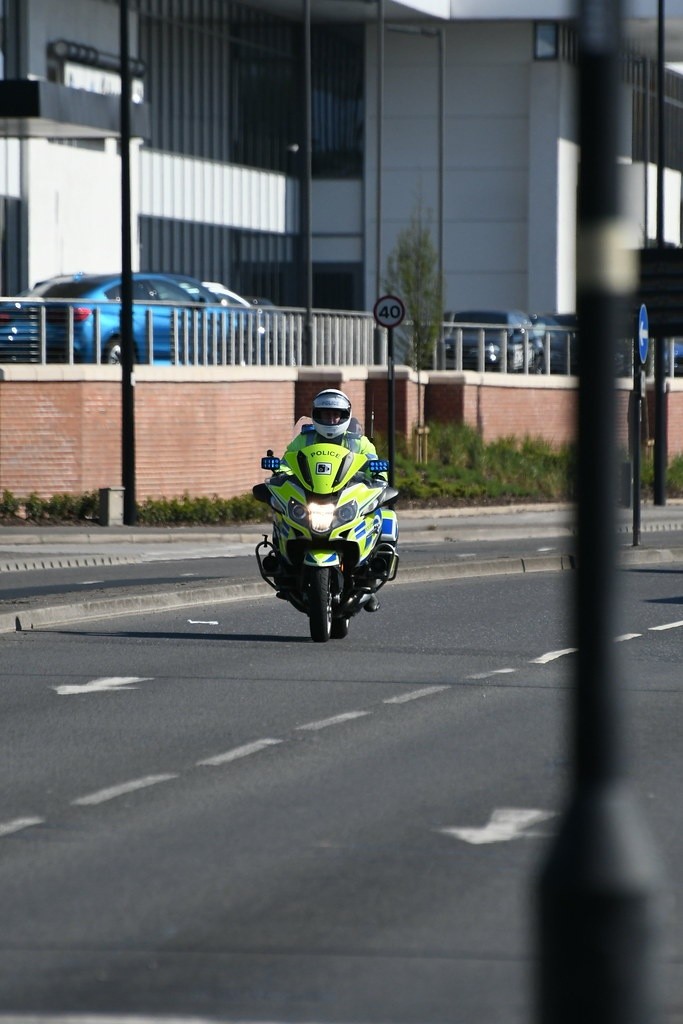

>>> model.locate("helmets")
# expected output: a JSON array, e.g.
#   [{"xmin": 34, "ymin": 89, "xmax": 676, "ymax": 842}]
[{"xmin": 311, "ymin": 389, "xmax": 351, "ymax": 440}]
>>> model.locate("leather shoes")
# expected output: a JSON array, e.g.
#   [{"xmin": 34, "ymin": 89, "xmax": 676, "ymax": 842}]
[
  {"xmin": 364, "ymin": 592, "xmax": 380, "ymax": 612},
  {"xmin": 263, "ymin": 556, "xmax": 280, "ymax": 585}
]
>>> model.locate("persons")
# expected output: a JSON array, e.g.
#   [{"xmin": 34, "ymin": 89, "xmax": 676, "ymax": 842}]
[{"xmin": 271, "ymin": 389, "xmax": 382, "ymax": 613}]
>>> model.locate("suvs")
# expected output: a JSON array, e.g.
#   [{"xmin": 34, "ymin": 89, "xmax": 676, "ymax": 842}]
[{"xmin": 430, "ymin": 306, "xmax": 546, "ymax": 378}]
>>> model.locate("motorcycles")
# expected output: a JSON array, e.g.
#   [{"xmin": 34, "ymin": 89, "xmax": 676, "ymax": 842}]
[{"xmin": 250, "ymin": 453, "xmax": 400, "ymax": 642}]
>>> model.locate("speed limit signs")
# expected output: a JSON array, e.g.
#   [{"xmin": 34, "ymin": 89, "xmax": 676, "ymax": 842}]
[{"xmin": 373, "ymin": 294, "xmax": 406, "ymax": 328}]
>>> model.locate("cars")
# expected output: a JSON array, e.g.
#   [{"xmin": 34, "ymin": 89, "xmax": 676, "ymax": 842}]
[
  {"xmin": 1, "ymin": 271, "xmax": 236, "ymax": 366},
  {"xmin": 164, "ymin": 271, "xmax": 309, "ymax": 368},
  {"xmin": 526, "ymin": 313, "xmax": 683, "ymax": 379}
]
